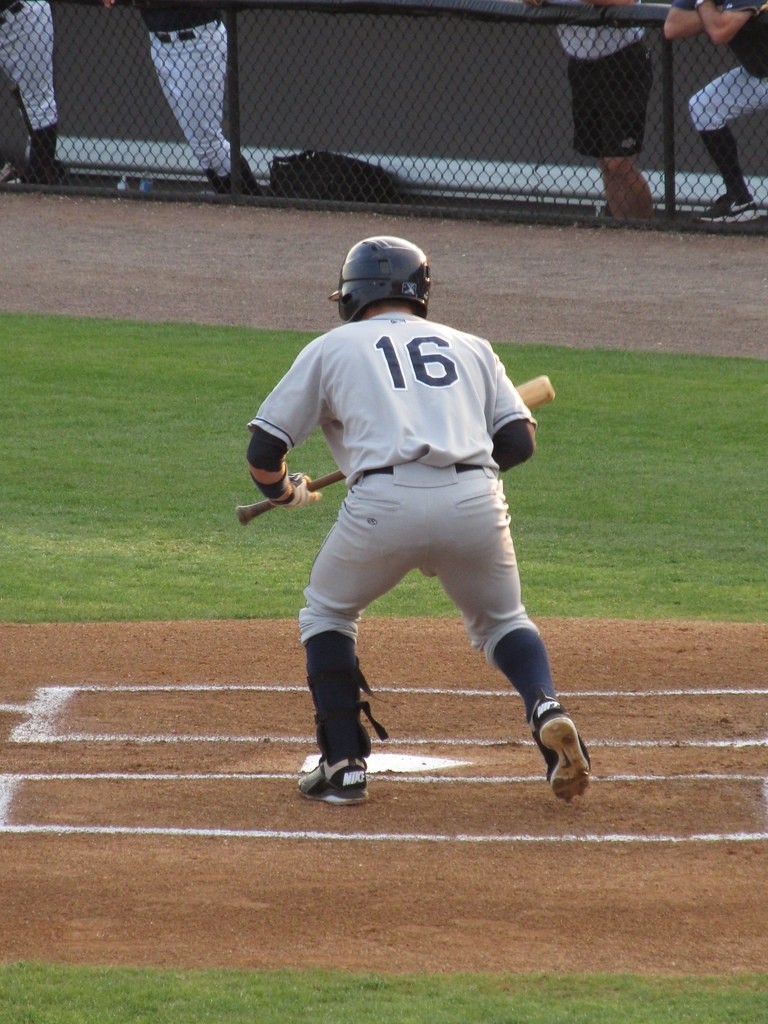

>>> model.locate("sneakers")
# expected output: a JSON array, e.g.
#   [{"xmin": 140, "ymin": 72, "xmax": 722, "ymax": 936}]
[
  {"xmin": 298, "ymin": 753, "xmax": 369, "ymax": 805},
  {"xmin": 696, "ymin": 192, "xmax": 760, "ymax": 222},
  {"xmin": 531, "ymin": 688, "xmax": 590, "ymax": 801}
]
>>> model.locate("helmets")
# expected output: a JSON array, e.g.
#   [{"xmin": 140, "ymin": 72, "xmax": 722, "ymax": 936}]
[{"xmin": 328, "ymin": 235, "xmax": 431, "ymax": 324}]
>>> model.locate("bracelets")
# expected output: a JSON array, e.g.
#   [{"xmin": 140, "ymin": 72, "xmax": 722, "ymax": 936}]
[{"xmin": 250, "ymin": 465, "xmax": 291, "ymax": 501}]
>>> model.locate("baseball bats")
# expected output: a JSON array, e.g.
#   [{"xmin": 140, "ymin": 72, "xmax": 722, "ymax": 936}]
[{"xmin": 236, "ymin": 373, "xmax": 557, "ymax": 515}]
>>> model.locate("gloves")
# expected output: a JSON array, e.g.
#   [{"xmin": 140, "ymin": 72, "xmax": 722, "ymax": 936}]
[
  {"xmin": 672, "ymin": 0, "xmax": 705, "ymax": 10},
  {"xmin": 269, "ymin": 472, "xmax": 323, "ymax": 510}
]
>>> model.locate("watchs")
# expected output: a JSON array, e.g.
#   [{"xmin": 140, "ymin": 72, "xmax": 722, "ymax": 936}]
[{"xmin": 694, "ymin": 0, "xmax": 704, "ymax": 9}]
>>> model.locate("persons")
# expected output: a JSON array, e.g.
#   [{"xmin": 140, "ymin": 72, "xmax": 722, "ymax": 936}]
[
  {"xmin": 0, "ymin": 0, "xmax": 65, "ymax": 191},
  {"xmin": 247, "ymin": 234, "xmax": 593, "ymax": 806},
  {"xmin": 104, "ymin": 1, "xmax": 265, "ymax": 197},
  {"xmin": 522, "ymin": 0, "xmax": 768, "ymax": 227}
]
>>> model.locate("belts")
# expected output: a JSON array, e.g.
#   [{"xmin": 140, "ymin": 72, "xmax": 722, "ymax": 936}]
[
  {"xmin": 158, "ymin": 20, "xmax": 222, "ymax": 43},
  {"xmin": 363, "ymin": 462, "xmax": 483, "ymax": 478},
  {"xmin": 0, "ymin": 2, "xmax": 24, "ymax": 25}
]
